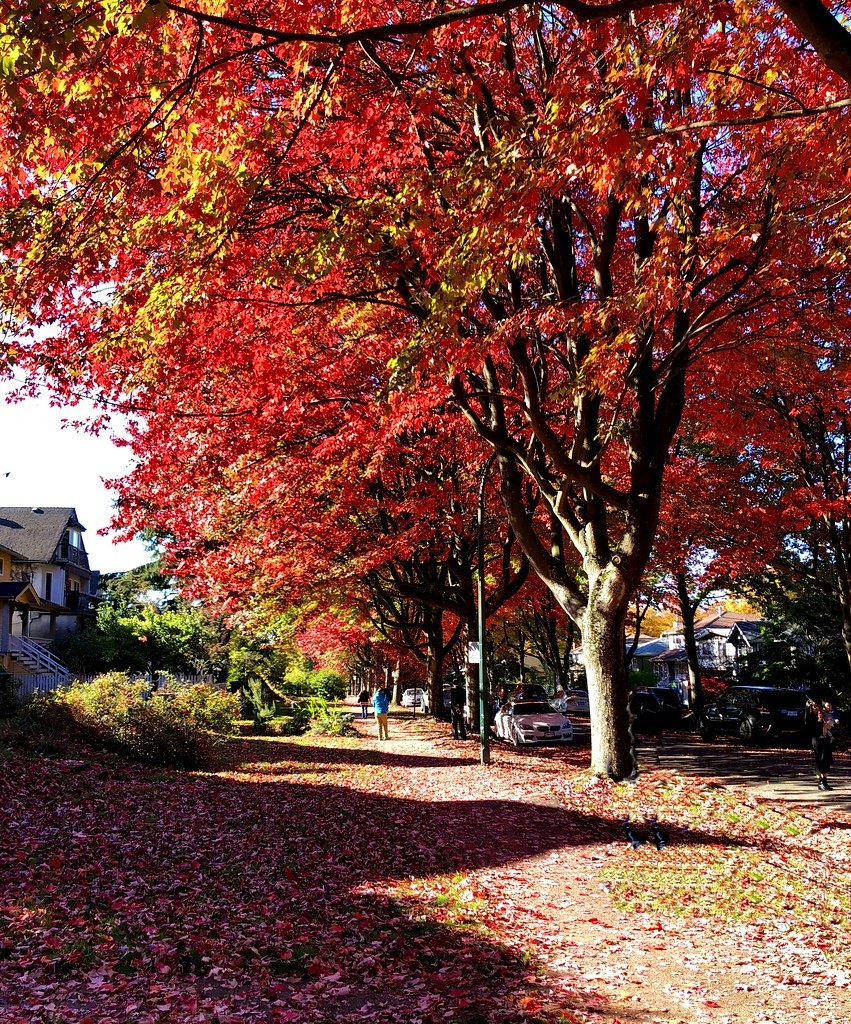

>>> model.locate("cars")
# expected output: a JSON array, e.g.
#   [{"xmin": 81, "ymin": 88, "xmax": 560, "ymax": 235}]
[
  {"xmin": 548, "ymin": 690, "xmax": 589, "ymax": 715},
  {"xmin": 636, "ymin": 687, "xmax": 684, "ymax": 719},
  {"xmin": 424, "ymin": 685, "xmax": 452, "ymax": 713},
  {"xmin": 401, "ymin": 688, "xmax": 424, "ymax": 707},
  {"xmin": 493, "ymin": 699, "xmax": 573, "ymax": 747}
]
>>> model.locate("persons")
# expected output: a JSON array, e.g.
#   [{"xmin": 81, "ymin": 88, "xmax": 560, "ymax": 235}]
[
  {"xmin": 819, "ymin": 699, "xmax": 841, "ymax": 744},
  {"xmin": 449, "ymin": 678, "xmax": 466, "ymax": 740},
  {"xmin": 805, "ymin": 699, "xmax": 835, "ymax": 791},
  {"xmin": 357, "ymin": 685, "xmax": 372, "ymax": 719},
  {"xmin": 371, "ymin": 687, "xmax": 390, "ymax": 741},
  {"xmin": 378, "ymin": 682, "xmax": 390, "ymax": 716}
]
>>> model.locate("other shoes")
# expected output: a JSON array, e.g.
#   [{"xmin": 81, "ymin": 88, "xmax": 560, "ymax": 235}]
[
  {"xmin": 385, "ymin": 737, "xmax": 389, "ymax": 740},
  {"xmin": 819, "ymin": 735, "xmax": 826, "ymax": 739},
  {"xmin": 824, "ymin": 783, "xmax": 833, "ymax": 790},
  {"xmin": 830, "ymin": 737, "xmax": 834, "ymax": 743},
  {"xmin": 818, "ymin": 784, "xmax": 827, "ymax": 791},
  {"xmin": 379, "ymin": 738, "xmax": 382, "ymax": 740}
]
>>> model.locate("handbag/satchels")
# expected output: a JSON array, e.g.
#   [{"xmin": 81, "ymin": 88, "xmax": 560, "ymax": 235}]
[{"xmin": 358, "ymin": 697, "xmax": 361, "ymax": 702}]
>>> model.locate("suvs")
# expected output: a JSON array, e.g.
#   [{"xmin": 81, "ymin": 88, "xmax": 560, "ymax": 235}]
[
  {"xmin": 490, "ymin": 684, "xmax": 516, "ymax": 709},
  {"xmin": 508, "ymin": 683, "xmax": 548, "ymax": 702},
  {"xmin": 699, "ymin": 686, "xmax": 814, "ymax": 746}
]
